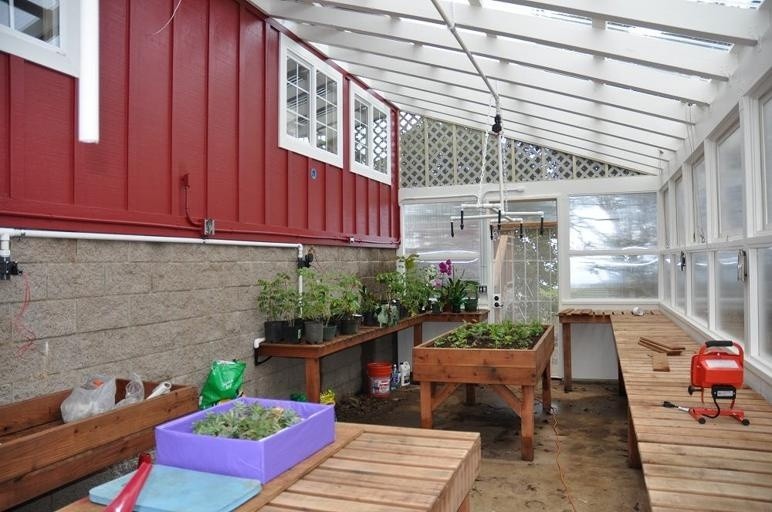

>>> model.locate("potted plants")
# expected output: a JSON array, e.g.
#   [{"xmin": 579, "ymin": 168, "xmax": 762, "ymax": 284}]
[
  {"xmin": 412, "ymin": 318, "xmax": 556, "ymax": 463},
  {"xmin": 261, "ymin": 253, "xmax": 481, "ymax": 343}
]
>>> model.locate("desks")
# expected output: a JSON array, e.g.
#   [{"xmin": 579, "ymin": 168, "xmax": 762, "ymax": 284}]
[
  {"xmin": 260, "ymin": 307, "xmax": 490, "ymax": 402},
  {"xmin": 557, "ymin": 306, "xmax": 771, "ymax": 512},
  {"xmin": 54, "ymin": 421, "xmax": 483, "ymax": 512}
]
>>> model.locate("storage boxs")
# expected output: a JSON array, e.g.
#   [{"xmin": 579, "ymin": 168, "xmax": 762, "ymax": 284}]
[{"xmin": 0, "ymin": 378, "xmax": 199, "ymax": 512}]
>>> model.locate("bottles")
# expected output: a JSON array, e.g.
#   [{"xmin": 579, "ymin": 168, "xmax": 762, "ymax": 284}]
[{"xmin": 391, "ymin": 363, "xmax": 402, "ymax": 390}]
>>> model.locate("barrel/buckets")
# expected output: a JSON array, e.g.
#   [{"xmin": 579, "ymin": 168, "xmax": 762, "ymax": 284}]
[
  {"xmin": 367, "ymin": 361, "xmax": 395, "ymax": 399},
  {"xmin": 399, "ymin": 361, "xmax": 411, "ymax": 386},
  {"xmin": 390, "ymin": 364, "xmax": 399, "ymax": 386}
]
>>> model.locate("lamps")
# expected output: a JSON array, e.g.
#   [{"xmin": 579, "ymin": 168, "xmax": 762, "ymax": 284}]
[{"xmin": 448, "ymin": 93, "xmax": 545, "ymax": 241}]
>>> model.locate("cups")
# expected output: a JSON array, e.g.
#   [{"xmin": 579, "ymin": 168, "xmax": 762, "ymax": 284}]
[{"xmin": 632, "ymin": 306, "xmax": 643, "ymax": 316}]
